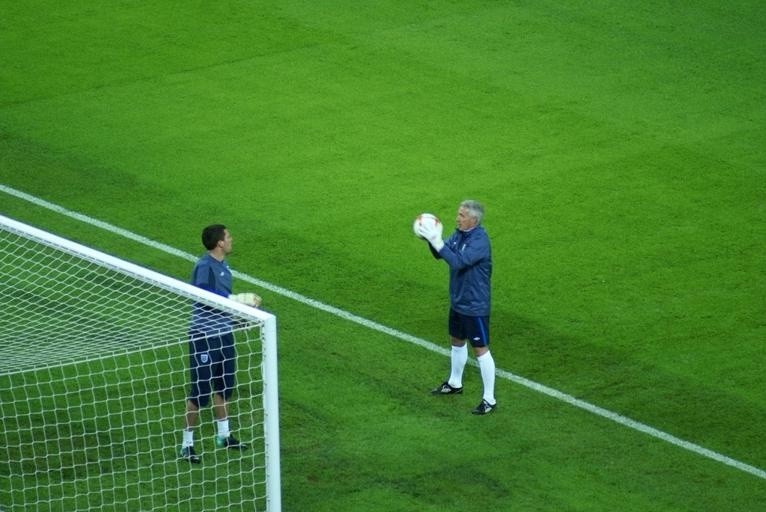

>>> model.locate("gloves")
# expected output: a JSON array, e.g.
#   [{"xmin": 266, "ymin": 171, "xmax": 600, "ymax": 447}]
[
  {"xmin": 228, "ymin": 292, "xmax": 262, "ymax": 308},
  {"xmin": 417, "ymin": 221, "xmax": 445, "ymax": 253}
]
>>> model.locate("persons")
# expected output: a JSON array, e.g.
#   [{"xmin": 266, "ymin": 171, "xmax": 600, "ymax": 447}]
[
  {"xmin": 179, "ymin": 224, "xmax": 262, "ymax": 463},
  {"xmin": 418, "ymin": 199, "xmax": 498, "ymax": 415}
]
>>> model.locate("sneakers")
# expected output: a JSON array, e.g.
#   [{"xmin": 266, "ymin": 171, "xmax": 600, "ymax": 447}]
[
  {"xmin": 179, "ymin": 445, "xmax": 201, "ymax": 465},
  {"xmin": 472, "ymin": 399, "xmax": 497, "ymax": 416},
  {"xmin": 430, "ymin": 381, "xmax": 463, "ymax": 396},
  {"xmin": 216, "ymin": 434, "xmax": 249, "ymax": 452}
]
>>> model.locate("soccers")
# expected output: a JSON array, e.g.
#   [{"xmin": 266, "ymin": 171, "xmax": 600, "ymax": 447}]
[{"xmin": 413, "ymin": 213, "xmax": 441, "ymax": 240}]
